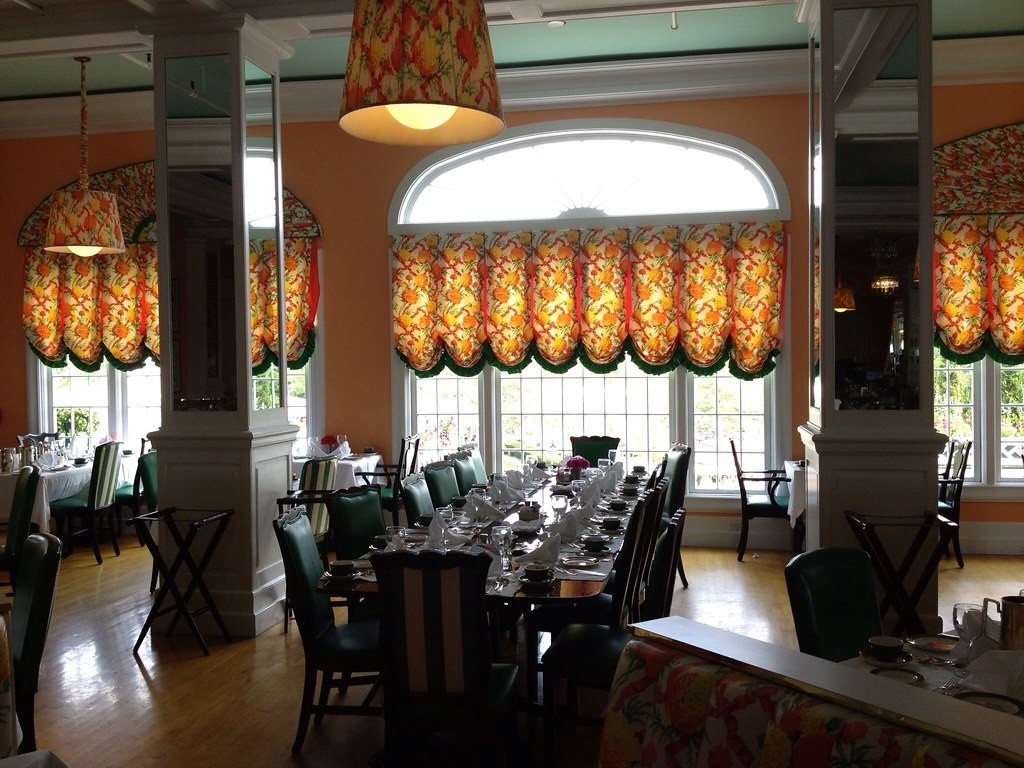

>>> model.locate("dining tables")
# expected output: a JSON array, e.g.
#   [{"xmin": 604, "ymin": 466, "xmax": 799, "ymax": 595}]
[
  {"xmin": 783, "ymin": 458, "xmax": 806, "ymax": 526},
  {"xmin": 840, "ymin": 619, "xmax": 1024, "ymax": 717},
  {"xmin": 0, "ymin": 449, "xmax": 142, "ymax": 532},
  {"xmin": 351, "ymin": 468, "xmax": 646, "ymax": 748},
  {"xmin": 292, "ymin": 452, "xmax": 384, "ymax": 487}
]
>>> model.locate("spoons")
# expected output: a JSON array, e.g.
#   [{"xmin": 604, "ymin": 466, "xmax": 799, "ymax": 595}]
[{"xmin": 497, "ymin": 578, "xmax": 509, "ymax": 592}]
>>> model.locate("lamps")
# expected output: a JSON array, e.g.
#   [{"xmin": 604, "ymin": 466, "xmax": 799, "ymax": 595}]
[
  {"xmin": 337, "ymin": 0, "xmax": 506, "ymax": 145},
  {"xmin": 44, "ymin": 56, "xmax": 125, "ymax": 256},
  {"xmin": 871, "ymin": 273, "xmax": 899, "ymax": 294},
  {"xmin": 835, "ymin": 277, "xmax": 856, "ymax": 313}
]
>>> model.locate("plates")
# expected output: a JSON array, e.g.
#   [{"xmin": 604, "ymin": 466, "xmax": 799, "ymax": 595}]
[
  {"xmin": 518, "ymin": 574, "xmax": 555, "ymax": 586},
  {"xmin": 413, "ymin": 522, "xmax": 433, "ymax": 529},
  {"xmin": 620, "ymin": 494, "xmax": 639, "ymax": 499},
  {"xmin": 362, "ymin": 452, "xmax": 377, "ymax": 456},
  {"xmin": 560, "ymin": 556, "xmax": 598, "ymax": 567},
  {"xmin": 871, "ymin": 667, "xmax": 923, "ymax": 684},
  {"xmin": 599, "ymin": 527, "xmax": 625, "ymax": 533},
  {"xmin": 862, "ymin": 651, "xmax": 914, "ymax": 668},
  {"xmin": 954, "ymin": 693, "xmax": 1024, "ymax": 717},
  {"xmin": 579, "ymin": 547, "xmax": 610, "ymax": 554},
  {"xmin": 608, "ymin": 507, "xmax": 629, "ymax": 513},
  {"xmin": 909, "ymin": 636, "xmax": 960, "ymax": 651}
]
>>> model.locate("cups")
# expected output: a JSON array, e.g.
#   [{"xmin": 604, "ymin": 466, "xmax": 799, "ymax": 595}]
[
  {"xmin": 525, "ymin": 563, "xmax": 553, "ymax": 581},
  {"xmin": 585, "ymin": 540, "xmax": 605, "ymax": 552},
  {"xmin": 626, "ymin": 476, "xmax": 638, "ymax": 484},
  {"xmin": 603, "ymin": 519, "xmax": 621, "ymax": 531},
  {"xmin": 611, "ymin": 502, "xmax": 626, "ymax": 511},
  {"xmin": 623, "ymin": 488, "xmax": 637, "ymax": 495},
  {"xmin": 420, "ymin": 514, "xmax": 433, "ymax": 526},
  {"xmin": 374, "ymin": 535, "xmax": 386, "ymax": 548},
  {"xmin": 869, "ymin": 637, "xmax": 903, "ymax": 658},
  {"xmin": 452, "ymin": 497, "xmax": 467, "ymax": 509},
  {"xmin": 75, "ymin": 458, "xmax": 85, "ymax": 464},
  {"xmin": 633, "ymin": 466, "xmax": 644, "ymax": 473},
  {"xmin": 364, "ymin": 447, "xmax": 374, "ymax": 452},
  {"xmin": 329, "ymin": 560, "xmax": 354, "ymax": 576},
  {"xmin": 535, "ymin": 462, "xmax": 546, "ymax": 470},
  {"xmin": 984, "ymin": 596, "xmax": 1024, "ymax": 651}
]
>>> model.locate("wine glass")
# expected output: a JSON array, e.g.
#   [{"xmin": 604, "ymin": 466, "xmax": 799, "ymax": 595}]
[
  {"xmin": 553, "ymin": 495, "xmax": 568, "ymax": 521},
  {"xmin": 572, "ymin": 479, "xmax": 586, "ymax": 509},
  {"xmin": 954, "ymin": 604, "xmax": 986, "ymax": 676},
  {"xmin": 598, "ymin": 460, "xmax": 611, "ymax": 477},
  {"xmin": 436, "ymin": 507, "xmax": 454, "ymax": 544},
  {"xmin": 308, "ymin": 436, "xmax": 318, "ymax": 459},
  {"xmin": 492, "ymin": 526, "xmax": 511, "ymax": 569},
  {"xmin": 493, "ymin": 474, "xmax": 507, "ymax": 508},
  {"xmin": 608, "ymin": 450, "xmax": 621, "ymax": 465},
  {"xmin": 337, "ymin": 435, "xmax": 346, "ymax": 446},
  {"xmin": 384, "ymin": 526, "xmax": 406, "ymax": 551},
  {"xmin": 517, "ymin": 464, "xmax": 529, "ymax": 495},
  {"xmin": 471, "ymin": 489, "xmax": 486, "ymax": 527}
]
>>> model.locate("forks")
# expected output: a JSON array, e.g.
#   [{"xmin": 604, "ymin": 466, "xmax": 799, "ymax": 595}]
[
  {"xmin": 941, "ymin": 677, "xmax": 958, "ymax": 693},
  {"xmin": 494, "ymin": 575, "xmax": 502, "ymax": 590}
]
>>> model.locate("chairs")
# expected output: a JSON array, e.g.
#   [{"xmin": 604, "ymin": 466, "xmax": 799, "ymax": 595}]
[
  {"xmin": 273, "ymin": 500, "xmax": 385, "ymax": 755},
  {"xmin": 297, "ymin": 457, "xmax": 338, "ymax": 571},
  {"xmin": 729, "ymin": 438, "xmax": 805, "ymax": 560},
  {"xmin": 398, "ymin": 470, "xmax": 436, "ymax": 528},
  {"xmin": 421, "ymin": 459, "xmax": 461, "ymax": 510},
  {"xmin": 570, "ymin": 435, "xmax": 620, "ymax": 467},
  {"xmin": 458, "ymin": 442, "xmax": 488, "ymax": 483},
  {"xmin": 17, "ymin": 431, "xmax": 60, "ymax": 459},
  {"xmin": 354, "ymin": 432, "xmax": 421, "ymax": 525},
  {"xmin": 320, "ymin": 483, "xmax": 394, "ymax": 691},
  {"xmin": 784, "ymin": 546, "xmax": 885, "ymax": 662},
  {"xmin": 444, "ymin": 450, "xmax": 478, "ymax": 496},
  {"xmin": 529, "ymin": 443, "xmax": 692, "ymax": 755},
  {"xmin": 938, "ymin": 437, "xmax": 972, "ymax": 567},
  {"xmin": 116, "ymin": 438, "xmax": 152, "ymax": 546},
  {"xmin": 11, "ymin": 531, "xmax": 63, "ymax": 757},
  {"xmin": 1, "ymin": 462, "xmax": 41, "ymax": 571},
  {"xmin": 50, "ymin": 440, "xmax": 125, "ymax": 564}
]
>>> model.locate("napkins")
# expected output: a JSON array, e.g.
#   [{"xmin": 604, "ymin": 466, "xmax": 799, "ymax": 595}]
[
  {"xmin": 427, "ymin": 456, "xmax": 623, "ymax": 561},
  {"xmin": 959, "ymin": 649, "xmax": 1024, "ymax": 714}
]
